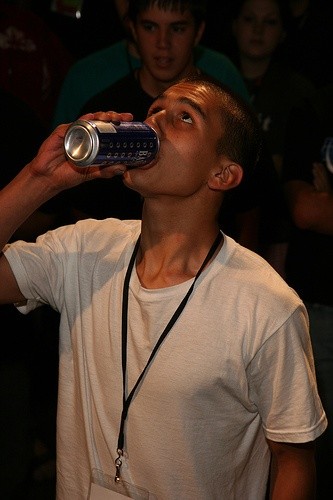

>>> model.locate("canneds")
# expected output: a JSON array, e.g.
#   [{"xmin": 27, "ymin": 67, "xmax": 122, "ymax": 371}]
[{"xmin": 63, "ymin": 121, "xmax": 159, "ymax": 167}]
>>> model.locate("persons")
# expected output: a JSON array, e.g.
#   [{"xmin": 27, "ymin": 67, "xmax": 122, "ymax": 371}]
[
  {"xmin": 1, "ymin": 74, "xmax": 328, "ymax": 500},
  {"xmin": 54, "ymin": 1, "xmax": 297, "ymax": 263}
]
